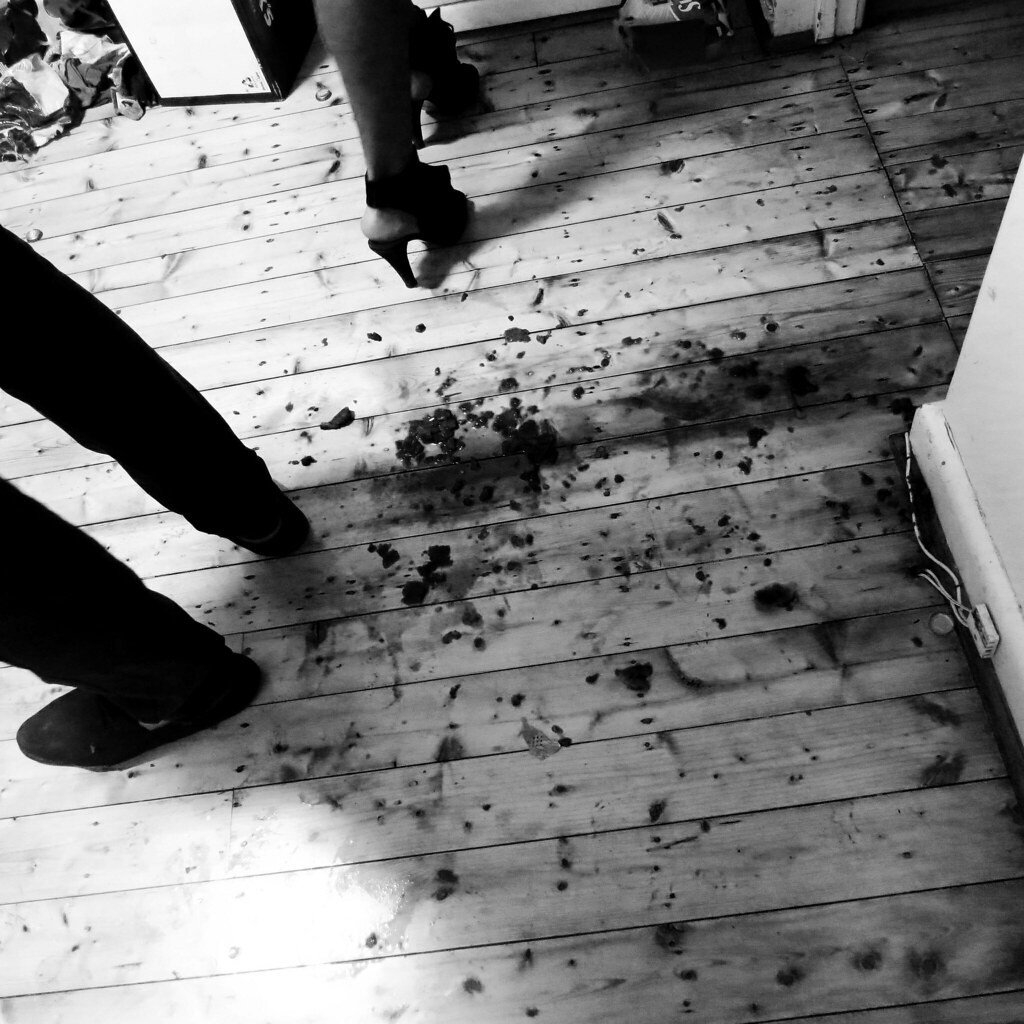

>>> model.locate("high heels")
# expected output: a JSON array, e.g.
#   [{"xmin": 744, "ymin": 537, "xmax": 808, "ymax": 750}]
[
  {"xmin": 363, "ymin": 166, "xmax": 470, "ymax": 289},
  {"xmin": 409, "ymin": 63, "xmax": 483, "ymax": 147}
]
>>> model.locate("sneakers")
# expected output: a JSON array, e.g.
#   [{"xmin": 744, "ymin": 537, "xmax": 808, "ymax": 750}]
[
  {"xmin": 16, "ymin": 651, "xmax": 263, "ymax": 766},
  {"xmin": 238, "ymin": 489, "xmax": 311, "ymax": 556}
]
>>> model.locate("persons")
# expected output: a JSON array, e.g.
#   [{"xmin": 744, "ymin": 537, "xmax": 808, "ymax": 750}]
[
  {"xmin": 0, "ymin": 224, "xmax": 313, "ymax": 769},
  {"xmin": 314, "ymin": 0, "xmax": 482, "ymax": 289}
]
